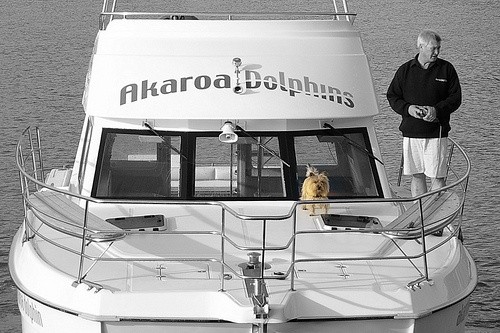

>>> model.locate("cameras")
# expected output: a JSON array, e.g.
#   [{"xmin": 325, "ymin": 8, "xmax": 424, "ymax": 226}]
[{"xmin": 415, "ymin": 107, "xmax": 428, "ymax": 117}]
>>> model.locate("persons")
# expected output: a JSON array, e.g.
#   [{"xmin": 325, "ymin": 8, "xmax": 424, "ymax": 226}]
[{"xmin": 387, "ymin": 31, "xmax": 462, "ymax": 236}]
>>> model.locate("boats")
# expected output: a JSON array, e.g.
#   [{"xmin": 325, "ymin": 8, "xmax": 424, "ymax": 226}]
[{"xmin": 8, "ymin": 0, "xmax": 478, "ymax": 332}]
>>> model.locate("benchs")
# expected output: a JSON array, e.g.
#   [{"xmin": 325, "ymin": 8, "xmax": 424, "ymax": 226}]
[
  {"xmin": 25, "ymin": 189, "xmax": 127, "ymax": 280},
  {"xmin": 381, "ymin": 186, "xmax": 461, "ymax": 277}
]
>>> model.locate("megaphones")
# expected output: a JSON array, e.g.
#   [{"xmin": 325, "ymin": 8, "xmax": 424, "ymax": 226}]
[{"xmin": 219, "ymin": 123, "xmax": 239, "ymax": 144}]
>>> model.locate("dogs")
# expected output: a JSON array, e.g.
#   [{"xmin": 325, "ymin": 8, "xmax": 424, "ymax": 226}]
[{"xmin": 298, "ymin": 161, "xmax": 330, "ymax": 216}]
